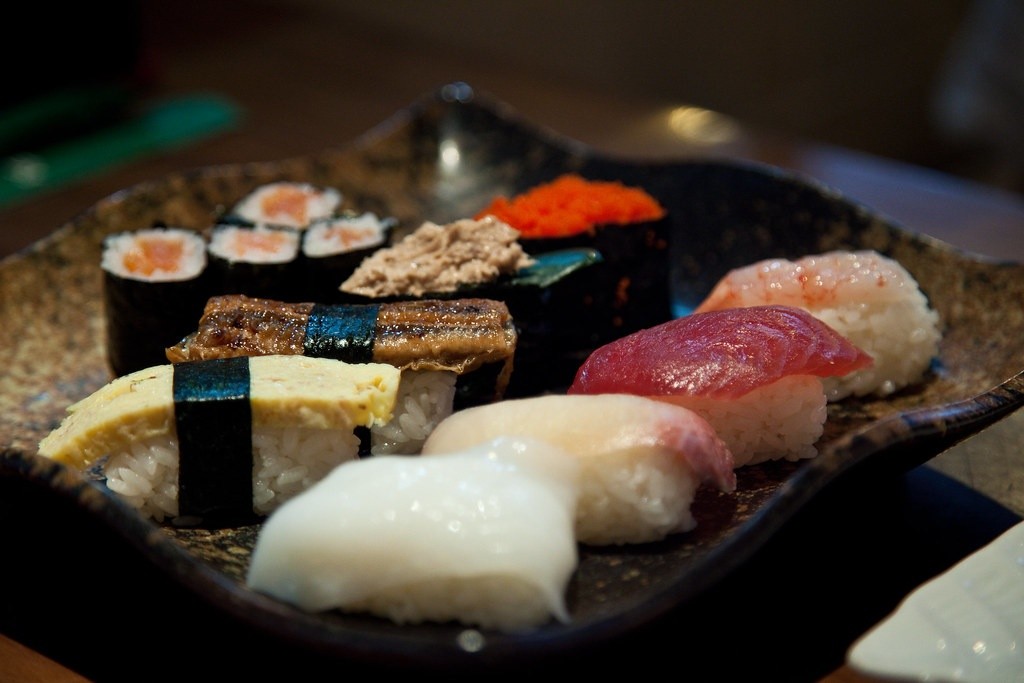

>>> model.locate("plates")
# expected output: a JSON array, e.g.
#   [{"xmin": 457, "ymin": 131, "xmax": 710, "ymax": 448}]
[{"xmin": 0, "ymin": 83, "xmax": 1024, "ymax": 683}]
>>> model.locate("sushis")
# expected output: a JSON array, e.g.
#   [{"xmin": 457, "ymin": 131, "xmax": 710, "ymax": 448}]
[{"xmin": 30, "ymin": 182, "xmax": 941, "ymax": 641}]
